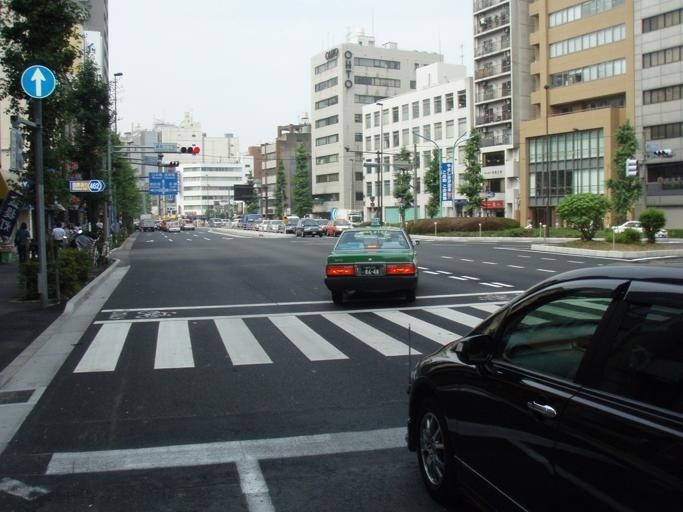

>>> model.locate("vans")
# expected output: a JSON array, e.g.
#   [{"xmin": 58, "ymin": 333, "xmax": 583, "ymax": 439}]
[{"xmin": 403, "ymin": 263, "xmax": 681, "ymax": 512}]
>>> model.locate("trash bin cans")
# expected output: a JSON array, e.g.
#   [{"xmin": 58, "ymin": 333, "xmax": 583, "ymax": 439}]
[
  {"xmin": 0, "ymin": 251, "xmax": 12, "ymax": 264},
  {"xmin": 542, "ymin": 224, "xmax": 549, "ymax": 237}
]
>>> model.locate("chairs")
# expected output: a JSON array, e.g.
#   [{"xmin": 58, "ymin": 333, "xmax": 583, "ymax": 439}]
[
  {"xmin": 346, "ymin": 241, "xmax": 364, "ymax": 248},
  {"xmin": 379, "ymin": 240, "xmax": 404, "ymax": 249}
]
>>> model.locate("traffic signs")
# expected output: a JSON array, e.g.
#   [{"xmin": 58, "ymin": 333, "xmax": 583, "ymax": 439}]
[
  {"xmin": 141, "ymin": 155, "xmax": 159, "ymax": 166},
  {"xmin": 148, "ymin": 172, "xmax": 179, "ymax": 196},
  {"xmin": 153, "ymin": 143, "xmax": 178, "ymax": 154}
]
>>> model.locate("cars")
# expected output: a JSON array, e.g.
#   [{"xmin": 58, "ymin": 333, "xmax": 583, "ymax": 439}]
[
  {"xmin": 295, "ymin": 217, "xmax": 323, "ymax": 237},
  {"xmin": 316, "ymin": 218, "xmax": 331, "ymax": 233},
  {"xmin": 206, "ymin": 213, "xmax": 301, "ymax": 234},
  {"xmin": 134, "ymin": 215, "xmax": 197, "ymax": 233},
  {"xmin": 324, "ymin": 224, "xmax": 420, "ymax": 305},
  {"xmin": 610, "ymin": 219, "xmax": 669, "ymax": 238},
  {"xmin": 326, "ymin": 216, "xmax": 353, "ymax": 237}
]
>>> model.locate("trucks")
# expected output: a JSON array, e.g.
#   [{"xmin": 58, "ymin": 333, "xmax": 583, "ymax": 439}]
[{"xmin": 329, "ymin": 207, "xmax": 363, "ymax": 226}]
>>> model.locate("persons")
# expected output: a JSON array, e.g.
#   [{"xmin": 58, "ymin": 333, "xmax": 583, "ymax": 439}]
[{"xmin": 14, "ymin": 219, "xmax": 104, "ymax": 262}]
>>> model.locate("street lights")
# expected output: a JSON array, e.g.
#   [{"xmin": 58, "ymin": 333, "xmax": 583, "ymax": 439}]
[
  {"xmin": 376, "ymin": 102, "xmax": 385, "ymax": 224},
  {"xmin": 343, "ymin": 143, "xmax": 380, "ymax": 224},
  {"xmin": 260, "ymin": 142, "xmax": 271, "ymax": 222},
  {"xmin": 114, "ymin": 72, "xmax": 127, "ymax": 142}
]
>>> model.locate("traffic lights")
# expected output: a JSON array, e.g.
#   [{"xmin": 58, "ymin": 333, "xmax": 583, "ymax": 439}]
[
  {"xmin": 626, "ymin": 157, "xmax": 639, "ymax": 178},
  {"xmin": 180, "ymin": 147, "xmax": 201, "ymax": 156},
  {"xmin": 653, "ymin": 148, "xmax": 674, "ymax": 159},
  {"xmin": 167, "ymin": 160, "xmax": 179, "ymax": 168}
]
